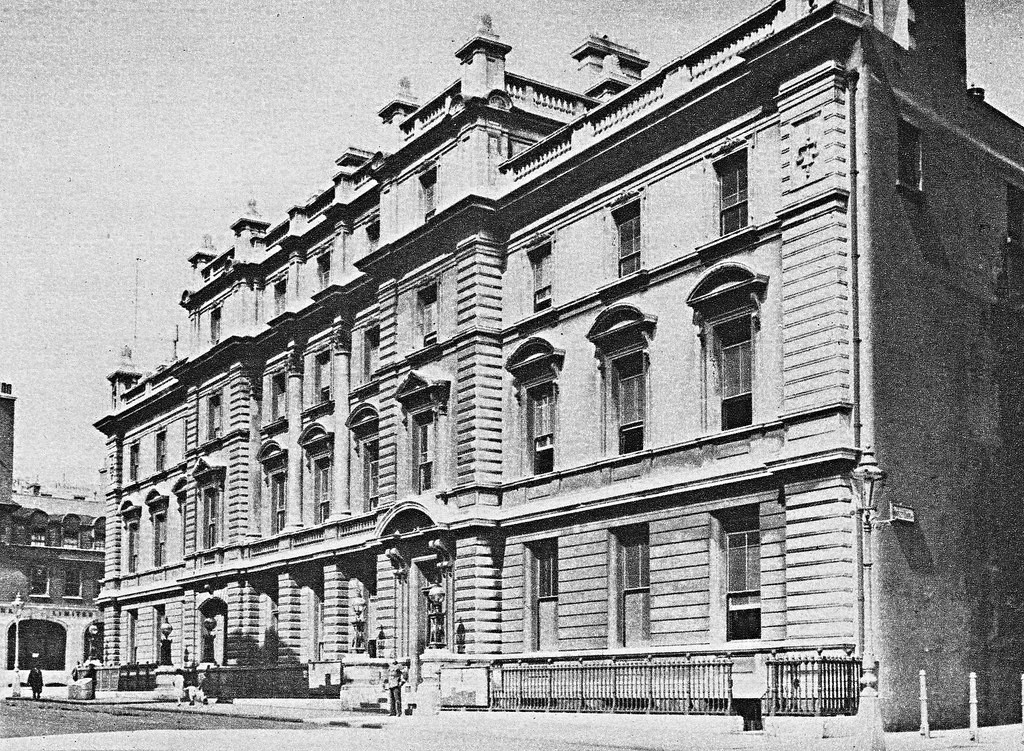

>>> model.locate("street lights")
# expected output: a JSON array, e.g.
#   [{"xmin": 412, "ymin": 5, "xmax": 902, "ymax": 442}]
[
  {"xmin": 850, "ymin": 443, "xmax": 888, "ymax": 751},
  {"xmin": 350, "ymin": 587, "xmax": 368, "ymax": 653},
  {"xmin": 87, "ymin": 620, "xmax": 99, "ymax": 659},
  {"xmin": 10, "ymin": 590, "xmax": 26, "ymax": 700},
  {"xmin": 201, "ymin": 618, "xmax": 218, "ymax": 662},
  {"xmin": 158, "ymin": 614, "xmax": 172, "ymax": 664},
  {"xmin": 425, "ymin": 587, "xmax": 449, "ymax": 650}
]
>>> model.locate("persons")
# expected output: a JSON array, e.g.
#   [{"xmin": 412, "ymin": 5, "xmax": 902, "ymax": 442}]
[
  {"xmin": 383, "ymin": 658, "xmax": 407, "ymax": 716},
  {"xmin": 172, "ymin": 668, "xmax": 207, "ymax": 706},
  {"xmin": 27, "ymin": 662, "xmax": 43, "ymax": 699},
  {"xmin": 83, "ymin": 664, "xmax": 96, "ymax": 700},
  {"xmin": 71, "ymin": 661, "xmax": 80, "ymax": 681}
]
